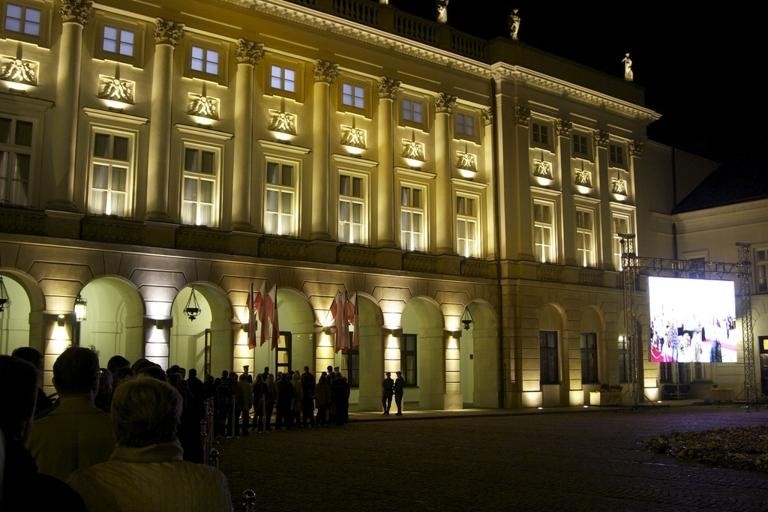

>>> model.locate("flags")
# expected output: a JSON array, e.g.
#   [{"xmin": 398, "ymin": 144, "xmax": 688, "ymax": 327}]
[
  {"xmin": 329, "ymin": 292, "xmax": 360, "ymax": 354},
  {"xmin": 246, "ymin": 282, "xmax": 279, "ymax": 351}
]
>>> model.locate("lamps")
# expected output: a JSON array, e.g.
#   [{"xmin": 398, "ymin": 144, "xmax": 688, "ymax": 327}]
[
  {"xmin": 461, "ymin": 307, "xmax": 472, "ymax": 330},
  {"xmin": 0, "ymin": 277, "xmax": 8, "ymax": 311},
  {"xmin": 183, "ymin": 288, "xmax": 201, "ymax": 321},
  {"xmin": 74, "ymin": 292, "xmax": 87, "ymax": 322}
]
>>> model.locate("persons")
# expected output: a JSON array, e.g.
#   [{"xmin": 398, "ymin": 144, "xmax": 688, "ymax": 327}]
[
  {"xmin": 65, "ymin": 373, "xmax": 233, "ymax": 512},
  {"xmin": 23, "ymin": 347, "xmax": 115, "ymax": 484},
  {"xmin": 2, "ymin": 355, "xmax": 87, "ymax": 512},
  {"xmin": 381, "ymin": 372, "xmax": 394, "ymax": 416},
  {"xmin": 94, "ymin": 356, "xmax": 349, "ymax": 460},
  {"xmin": 622, "ymin": 53, "xmax": 633, "ymax": 80},
  {"xmin": 11, "ymin": 347, "xmax": 52, "ymax": 418},
  {"xmin": 508, "ymin": 7, "xmax": 522, "ymax": 39},
  {"xmin": 393, "ymin": 370, "xmax": 403, "ymax": 415},
  {"xmin": 435, "ymin": 0, "xmax": 449, "ymax": 23}
]
